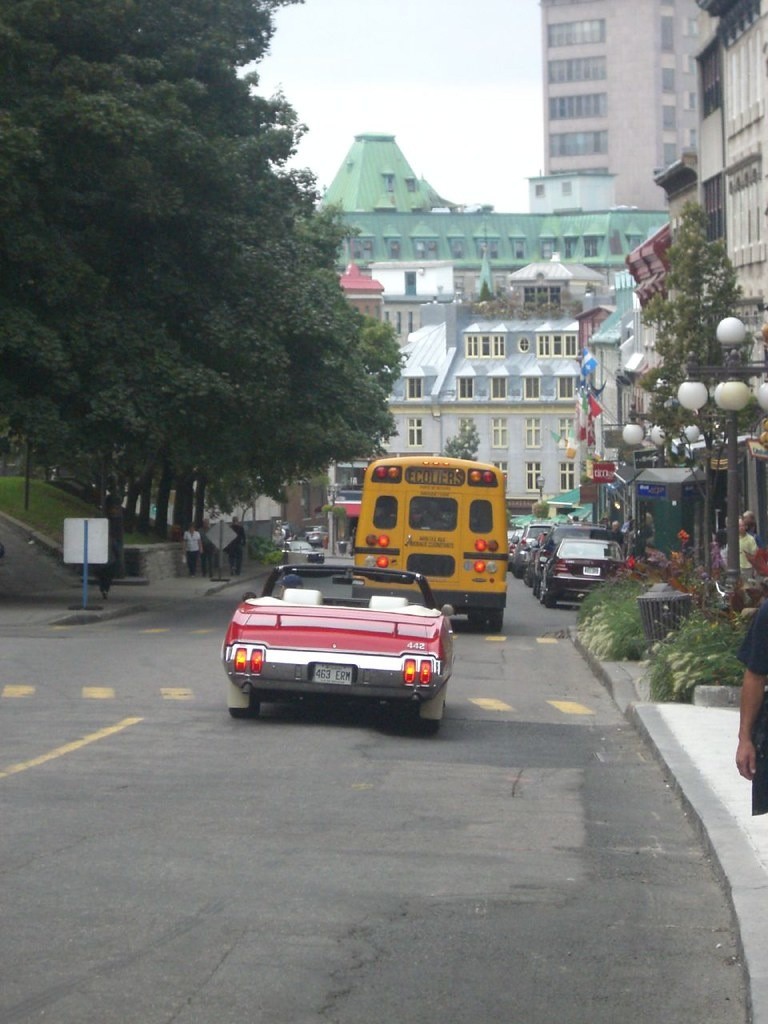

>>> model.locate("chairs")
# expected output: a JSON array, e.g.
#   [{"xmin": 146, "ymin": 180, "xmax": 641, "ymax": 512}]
[
  {"xmin": 283, "ymin": 588, "xmax": 323, "ymax": 605},
  {"xmin": 369, "ymin": 595, "xmax": 408, "ymax": 613}
]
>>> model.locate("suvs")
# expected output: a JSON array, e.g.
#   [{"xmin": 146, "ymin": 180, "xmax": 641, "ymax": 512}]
[
  {"xmin": 273, "ymin": 521, "xmax": 298, "ymax": 543},
  {"xmin": 535, "ymin": 521, "xmax": 618, "ymax": 599},
  {"xmin": 511, "ymin": 523, "xmax": 552, "ymax": 578}
]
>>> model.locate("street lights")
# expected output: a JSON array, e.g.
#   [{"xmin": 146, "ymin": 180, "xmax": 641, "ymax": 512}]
[
  {"xmin": 677, "ymin": 314, "xmax": 768, "ymax": 600},
  {"xmin": 624, "ymin": 393, "xmax": 699, "ymax": 470},
  {"xmin": 536, "ymin": 476, "xmax": 545, "ymax": 504}
]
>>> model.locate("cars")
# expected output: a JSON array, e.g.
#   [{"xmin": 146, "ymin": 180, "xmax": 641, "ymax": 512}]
[
  {"xmin": 508, "ymin": 524, "xmax": 519, "ymax": 542},
  {"xmin": 541, "ymin": 540, "xmax": 628, "ymax": 606},
  {"xmin": 526, "ymin": 538, "xmax": 548, "ymax": 586},
  {"xmin": 281, "ymin": 539, "xmax": 325, "ymax": 565},
  {"xmin": 305, "ymin": 526, "xmax": 328, "ymax": 547}
]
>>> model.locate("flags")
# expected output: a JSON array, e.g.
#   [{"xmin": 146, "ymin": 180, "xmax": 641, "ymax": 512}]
[
  {"xmin": 567, "ymin": 381, "xmax": 606, "ymax": 458},
  {"xmin": 580, "ymin": 349, "xmax": 599, "ymax": 377},
  {"xmin": 552, "ymin": 432, "xmax": 567, "ymax": 447}
]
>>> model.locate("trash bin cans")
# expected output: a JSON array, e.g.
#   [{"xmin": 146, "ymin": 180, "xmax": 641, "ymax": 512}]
[{"xmin": 637, "ymin": 580, "xmax": 693, "ymax": 643}]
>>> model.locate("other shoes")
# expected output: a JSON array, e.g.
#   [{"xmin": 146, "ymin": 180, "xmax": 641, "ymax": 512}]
[
  {"xmin": 190, "ymin": 573, "xmax": 195, "ymax": 577},
  {"xmin": 236, "ymin": 570, "xmax": 240, "ymax": 575},
  {"xmin": 230, "ymin": 570, "xmax": 233, "ymax": 576}
]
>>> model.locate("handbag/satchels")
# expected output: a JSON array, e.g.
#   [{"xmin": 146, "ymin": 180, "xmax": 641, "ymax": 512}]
[{"xmin": 183, "ymin": 555, "xmax": 187, "ymax": 563}]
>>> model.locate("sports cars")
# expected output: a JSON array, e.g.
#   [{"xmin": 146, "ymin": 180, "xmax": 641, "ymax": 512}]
[{"xmin": 222, "ymin": 565, "xmax": 456, "ymax": 734}]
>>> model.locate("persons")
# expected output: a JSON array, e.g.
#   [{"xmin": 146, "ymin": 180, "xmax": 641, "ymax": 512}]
[
  {"xmin": 736, "ymin": 598, "xmax": 768, "ymax": 816},
  {"xmin": 570, "ymin": 510, "xmax": 763, "ymax": 581},
  {"xmin": 198, "ymin": 519, "xmax": 216, "ymax": 576},
  {"xmin": 183, "ymin": 522, "xmax": 203, "ymax": 577},
  {"xmin": 224, "ymin": 516, "xmax": 247, "ymax": 576},
  {"xmin": 95, "ymin": 539, "xmax": 122, "ymax": 600},
  {"xmin": 275, "ymin": 574, "xmax": 305, "ymax": 599}
]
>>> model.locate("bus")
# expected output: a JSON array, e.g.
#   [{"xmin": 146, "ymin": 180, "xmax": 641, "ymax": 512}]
[{"xmin": 353, "ymin": 458, "xmax": 510, "ymax": 632}]
